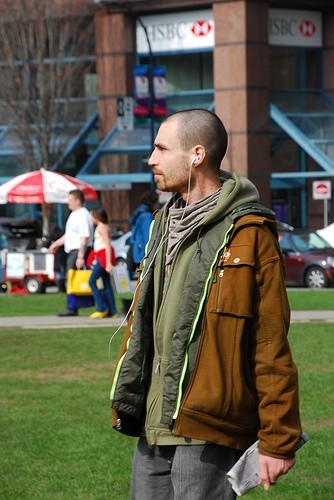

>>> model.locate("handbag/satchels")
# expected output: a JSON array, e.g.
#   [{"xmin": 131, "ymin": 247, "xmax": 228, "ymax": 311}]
[
  {"xmin": 67, "ymin": 265, "xmax": 99, "ymax": 295},
  {"xmin": 127, "ymin": 247, "xmax": 137, "ymax": 272}
]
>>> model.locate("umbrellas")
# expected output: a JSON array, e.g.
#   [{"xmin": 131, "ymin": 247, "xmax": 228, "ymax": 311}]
[{"xmin": 0, "ymin": 167, "xmax": 98, "ymax": 237}]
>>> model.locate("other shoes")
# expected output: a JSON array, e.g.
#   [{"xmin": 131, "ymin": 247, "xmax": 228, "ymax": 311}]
[
  {"xmin": 110, "ymin": 312, "xmax": 119, "ymax": 318},
  {"xmin": 90, "ymin": 311, "xmax": 108, "ymax": 318},
  {"xmin": 58, "ymin": 309, "xmax": 79, "ymax": 316}
]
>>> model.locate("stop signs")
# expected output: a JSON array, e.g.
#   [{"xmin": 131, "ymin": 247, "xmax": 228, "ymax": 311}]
[{"xmin": 315, "ymin": 184, "xmax": 329, "ymax": 196}]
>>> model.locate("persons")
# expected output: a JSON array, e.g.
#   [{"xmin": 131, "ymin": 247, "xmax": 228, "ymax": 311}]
[
  {"xmin": 48, "ymin": 189, "xmax": 95, "ymax": 317},
  {"xmin": 86, "ymin": 208, "xmax": 118, "ymax": 319},
  {"xmin": 127, "ymin": 192, "xmax": 157, "ymax": 269},
  {"xmin": 108, "ymin": 108, "xmax": 304, "ymax": 500}
]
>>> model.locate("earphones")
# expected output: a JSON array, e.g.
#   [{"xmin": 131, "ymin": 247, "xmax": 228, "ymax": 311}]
[{"xmin": 191, "ymin": 156, "xmax": 199, "ymax": 165}]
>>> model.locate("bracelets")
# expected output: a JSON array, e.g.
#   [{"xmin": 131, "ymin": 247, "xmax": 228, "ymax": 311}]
[{"xmin": 77, "ymin": 256, "xmax": 84, "ymax": 259}]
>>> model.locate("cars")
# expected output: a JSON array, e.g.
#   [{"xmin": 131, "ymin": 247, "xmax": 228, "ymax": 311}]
[
  {"xmin": 277, "ymin": 227, "xmax": 334, "ymax": 289},
  {"xmin": 110, "ymin": 229, "xmax": 135, "ymax": 268}
]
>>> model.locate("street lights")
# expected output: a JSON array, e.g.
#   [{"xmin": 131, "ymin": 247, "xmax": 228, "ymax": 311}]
[{"xmin": 93, "ymin": 0, "xmax": 158, "ymax": 188}]
